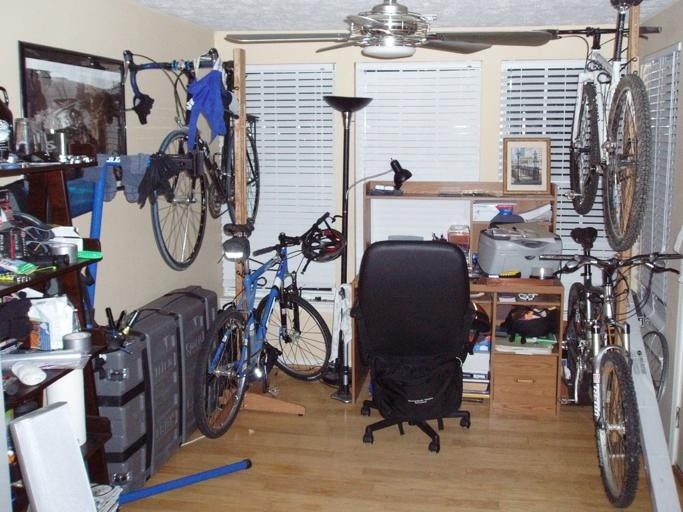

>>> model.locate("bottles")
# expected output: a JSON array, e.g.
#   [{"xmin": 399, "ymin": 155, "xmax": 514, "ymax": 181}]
[{"xmin": 11, "ymin": 118, "xmax": 34, "ymax": 158}]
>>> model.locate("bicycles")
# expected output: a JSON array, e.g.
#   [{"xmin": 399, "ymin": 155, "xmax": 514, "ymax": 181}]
[
  {"xmin": 550, "ymin": 0, "xmax": 661, "ymax": 251},
  {"xmin": 538, "ymin": 226, "xmax": 682, "ymax": 509},
  {"xmin": 120, "ymin": 44, "xmax": 260, "ymax": 270},
  {"xmin": 192, "ymin": 211, "xmax": 347, "ymax": 438}
]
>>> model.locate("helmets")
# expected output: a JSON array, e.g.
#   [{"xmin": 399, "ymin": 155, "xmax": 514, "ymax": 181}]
[
  {"xmin": 301, "ymin": 229, "xmax": 346, "ymax": 261},
  {"xmin": 472, "ymin": 304, "xmax": 490, "ymax": 332}
]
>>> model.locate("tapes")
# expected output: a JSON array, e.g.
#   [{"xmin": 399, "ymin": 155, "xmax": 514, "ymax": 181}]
[
  {"xmin": 62, "ymin": 332, "xmax": 93, "ymax": 355},
  {"xmin": 50, "ymin": 243, "xmax": 78, "ymax": 264}
]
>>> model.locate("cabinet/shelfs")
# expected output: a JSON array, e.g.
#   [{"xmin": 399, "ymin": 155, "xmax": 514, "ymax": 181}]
[{"xmin": 0, "ymin": 158, "xmax": 115, "ymax": 512}]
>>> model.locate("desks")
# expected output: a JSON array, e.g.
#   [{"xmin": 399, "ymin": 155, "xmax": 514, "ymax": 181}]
[{"xmin": 347, "ymin": 175, "xmax": 565, "ymax": 426}]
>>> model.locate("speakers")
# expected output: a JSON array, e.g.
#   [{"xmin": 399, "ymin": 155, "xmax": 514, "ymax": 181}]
[
  {"xmin": 42, "ymin": 366, "xmax": 87, "ymax": 447},
  {"xmin": 10, "ymin": 400, "xmax": 96, "ymax": 512}
]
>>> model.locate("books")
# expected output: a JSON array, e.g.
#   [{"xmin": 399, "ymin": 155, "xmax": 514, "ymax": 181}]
[
  {"xmin": 496, "ymin": 222, "xmax": 520, "ymax": 237},
  {"xmin": 88, "ymin": 482, "xmax": 123, "ymax": 511},
  {"xmin": 490, "ymin": 227, "xmax": 521, "ymax": 237},
  {"xmin": 461, "ymin": 351, "xmax": 490, "ymax": 395},
  {"xmin": 512, "ymin": 221, "xmax": 556, "ymax": 245},
  {"xmin": 493, "ymin": 328, "xmax": 557, "ymax": 355}
]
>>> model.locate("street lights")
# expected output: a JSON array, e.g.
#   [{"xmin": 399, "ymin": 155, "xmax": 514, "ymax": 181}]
[{"xmin": 516, "ymin": 148, "xmax": 521, "ymax": 183}]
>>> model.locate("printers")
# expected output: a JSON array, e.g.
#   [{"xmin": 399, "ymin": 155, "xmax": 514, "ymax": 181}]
[{"xmin": 476, "ymin": 222, "xmax": 563, "ymax": 278}]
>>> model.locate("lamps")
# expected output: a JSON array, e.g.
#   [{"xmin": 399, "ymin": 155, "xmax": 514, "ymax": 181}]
[
  {"xmin": 357, "ymin": 41, "xmax": 418, "ymax": 61},
  {"xmin": 319, "ymin": 94, "xmax": 414, "ymax": 387}
]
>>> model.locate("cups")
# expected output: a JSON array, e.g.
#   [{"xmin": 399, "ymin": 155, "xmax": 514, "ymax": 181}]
[{"xmin": 51, "ymin": 132, "xmax": 67, "ymax": 160}]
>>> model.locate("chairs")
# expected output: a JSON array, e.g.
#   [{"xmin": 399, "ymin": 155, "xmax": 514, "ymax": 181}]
[{"xmin": 347, "ymin": 237, "xmax": 491, "ymax": 456}]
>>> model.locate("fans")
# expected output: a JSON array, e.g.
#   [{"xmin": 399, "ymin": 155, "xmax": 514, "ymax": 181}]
[{"xmin": 218, "ymin": 1, "xmax": 564, "ymax": 69}]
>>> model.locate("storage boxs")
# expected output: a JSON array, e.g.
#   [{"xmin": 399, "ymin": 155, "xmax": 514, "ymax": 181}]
[{"xmin": 90, "ymin": 281, "xmax": 225, "ymax": 492}]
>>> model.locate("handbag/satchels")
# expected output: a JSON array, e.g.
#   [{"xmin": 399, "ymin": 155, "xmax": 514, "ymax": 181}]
[
  {"xmin": 372, "ymin": 354, "xmax": 462, "ymax": 423},
  {"xmin": 506, "ymin": 308, "xmax": 552, "ymax": 336}
]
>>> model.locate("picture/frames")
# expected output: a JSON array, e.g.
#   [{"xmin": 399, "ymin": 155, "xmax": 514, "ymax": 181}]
[
  {"xmin": 500, "ymin": 134, "xmax": 553, "ymax": 196},
  {"xmin": 15, "ymin": 38, "xmax": 130, "ymax": 193}
]
